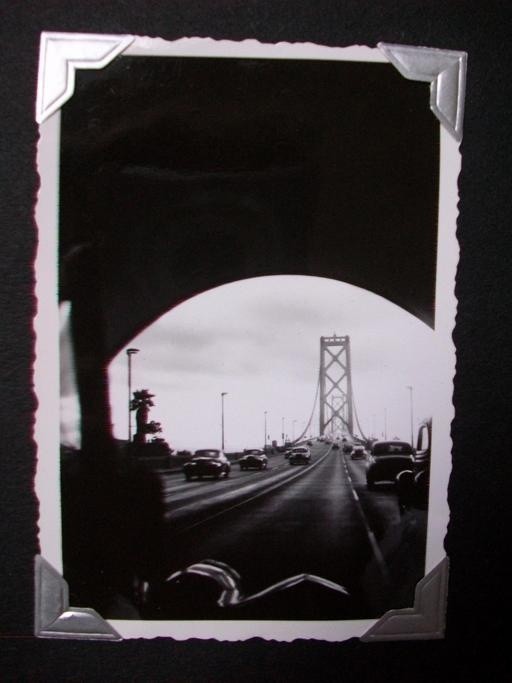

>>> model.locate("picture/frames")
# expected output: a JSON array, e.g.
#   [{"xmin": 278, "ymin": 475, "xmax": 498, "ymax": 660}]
[{"xmin": 31, "ymin": 32, "xmax": 470, "ymax": 645}]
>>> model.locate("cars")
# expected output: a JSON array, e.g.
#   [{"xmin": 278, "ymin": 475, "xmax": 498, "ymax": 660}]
[
  {"xmin": 365, "ymin": 415, "xmax": 430, "ymax": 519},
  {"xmin": 238, "ymin": 449, "xmax": 268, "ymax": 471},
  {"xmin": 317, "ymin": 435, "xmax": 367, "ymax": 459},
  {"xmin": 184, "ymin": 449, "xmax": 231, "ymax": 481},
  {"xmin": 285, "ymin": 440, "xmax": 314, "ymax": 464}
]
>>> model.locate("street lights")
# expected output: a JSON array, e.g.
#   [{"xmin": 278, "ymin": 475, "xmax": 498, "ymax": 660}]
[
  {"xmin": 126, "ymin": 348, "xmax": 139, "ymax": 440},
  {"xmin": 221, "ymin": 392, "xmax": 227, "ymax": 451}
]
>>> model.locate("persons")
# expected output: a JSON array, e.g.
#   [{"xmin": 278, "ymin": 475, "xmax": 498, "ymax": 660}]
[{"xmin": 59, "ymin": 455, "xmax": 169, "ymax": 619}]
